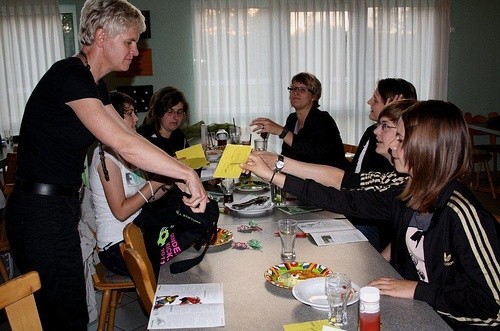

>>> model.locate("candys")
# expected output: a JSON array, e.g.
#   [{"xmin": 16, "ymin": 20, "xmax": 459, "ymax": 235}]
[{"xmin": 220, "ymin": 220, "xmax": 262, "ymax": 249}]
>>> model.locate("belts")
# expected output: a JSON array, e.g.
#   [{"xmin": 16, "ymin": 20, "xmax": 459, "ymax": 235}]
[{"xmin": 11, "ymin": 179, "xmax": 80, "ymax": 201}]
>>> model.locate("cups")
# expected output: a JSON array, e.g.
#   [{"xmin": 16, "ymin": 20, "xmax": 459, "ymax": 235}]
[
  {"xmin": 271, "ymin": 184, "xmax": 286, "ymax": 205},
  {"xmin": 254, "ymin": 139, "xmax": 267, "ymax": 151},
  {"xmin": 278, "ymin": 219, "xmax": 298, "ymax": 261},
  {"xmin": 220, "ymin": 178, "xmax": 234, "ymax": 204},
  {"xmin": 4, "ymin": 130, "xmax": 13, "ymax": 148},
  {"xmin": 229, "ymin": 125, "xmax": 241, "ymax": 144},
  {"xmin": 246, "ymin": 123, "xmax": 264, "ymax": 133},
  {"xmin": 324, "ymin": 273, "xmax": 351, "ymax": 326},
  {"xmin": 207, "ymin": 132, "xmax": 215, "ymax": 147}
]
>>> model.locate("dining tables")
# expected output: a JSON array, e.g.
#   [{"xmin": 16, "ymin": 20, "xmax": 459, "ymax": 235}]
[{"xmin": 156, "ymin": 214, "xmax": 455, "ymax": 330}]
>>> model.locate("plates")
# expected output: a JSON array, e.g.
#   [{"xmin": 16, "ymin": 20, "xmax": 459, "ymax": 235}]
[
  {"xmin": 225, "ymin": 197, "xmax": 275, "ymax": 215},
  {"xmin": 192, "ymin": 228, "xmax": 235, "ymax": 248},
  {"xmin": 291, "ymin": 277, "xmax": 360, "ymax": 311},
  {"xmin": 264, "ymin": 261, "xmax": 333, "ymax": 290},
  {"xmin": 235, "ymin": 181, "xmax": 269, "ymax": 191}
]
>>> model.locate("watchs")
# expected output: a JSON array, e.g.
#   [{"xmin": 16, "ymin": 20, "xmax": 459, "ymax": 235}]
[{"xmin": 275, "ymin": 155, "xmax": 285, "ymax": 171}]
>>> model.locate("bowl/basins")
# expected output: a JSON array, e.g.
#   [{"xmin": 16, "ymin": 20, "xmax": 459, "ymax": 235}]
[{"xmin": 205, "ymin": 151, "xmax": 220, "ymax": 160}]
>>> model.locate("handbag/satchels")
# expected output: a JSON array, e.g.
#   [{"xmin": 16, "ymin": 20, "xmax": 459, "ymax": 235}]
[{"xmin": 132, "ymin": 184, "xmax": 220, "ymax": 273}]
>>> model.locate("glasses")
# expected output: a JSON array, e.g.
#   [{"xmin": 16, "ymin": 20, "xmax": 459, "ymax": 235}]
[
  {"xmin": 287, "ymin": 86, "xmax": 316, "ymax": 95},
  {"xmin": 375, "ymin": 122, "xmax": 397, "ymax": 130},
  {"xmin": 166, "ymin": 109, "xmax": 185, "ymax": 116}
]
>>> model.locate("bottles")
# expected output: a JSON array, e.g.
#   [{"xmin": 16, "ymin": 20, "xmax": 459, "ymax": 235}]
[{"xmin": 359, "ymin": 286, "xmax": 380, "ymax": 331}]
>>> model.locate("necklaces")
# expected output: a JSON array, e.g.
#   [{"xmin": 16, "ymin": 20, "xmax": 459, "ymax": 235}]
[{"xmin": 79, "ymin": 49, "xmax": 91, "ymax": 70}]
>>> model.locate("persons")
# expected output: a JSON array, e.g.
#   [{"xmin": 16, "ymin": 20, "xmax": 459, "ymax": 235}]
[
  {"xmin": 90, "ymin": 91, "xmax": 174, "ymax": 276},
  {"xmin": 250, "ymin": 73, "xmax": 347, "ymax": 172},
  {"xmin": 138, "ymin": 85, "xmax": 201, "ymax": 178},
  {"xmin": 240, "ymin": 77, "xmax": 500, "ymax": 331},
  {"xmin": 5, "ymin": 0, "xmax": 211, "ymax": 331}
]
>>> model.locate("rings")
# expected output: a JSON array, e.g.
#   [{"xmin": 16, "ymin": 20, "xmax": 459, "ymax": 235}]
[{"xmin": 262, "ymin": 125, "xmax": 265, "ymax": 130}]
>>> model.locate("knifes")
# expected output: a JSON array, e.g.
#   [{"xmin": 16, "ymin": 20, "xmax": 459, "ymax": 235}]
[{"xmin": 231, "ymin": 196, "xmax": 270, "ymax": 210}]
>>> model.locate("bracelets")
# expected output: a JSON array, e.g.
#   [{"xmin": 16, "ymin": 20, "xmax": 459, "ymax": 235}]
[
  {"xmin": 279, "ymin": 127, "xmax": 289, "ymax": 139},
  {"xmin": 269, "ymin": 172, "xmax": 277, "ymax": 183},
  {"xmin": 137, "ymin": 190, "xmax": 149, "ymax": 203},
  {"xmin": 148, "ymin": 180, "xmax": 155, "ymax": 197}
]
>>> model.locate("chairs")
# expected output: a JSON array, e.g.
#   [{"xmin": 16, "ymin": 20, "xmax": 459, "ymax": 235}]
[
  {"xmin": 78, "ymin": 214, "xmax": 157, "ymax": 331},
  {"xmin": 462, "ymin": 109, "xmax": 500, "ymax": 202}
]
